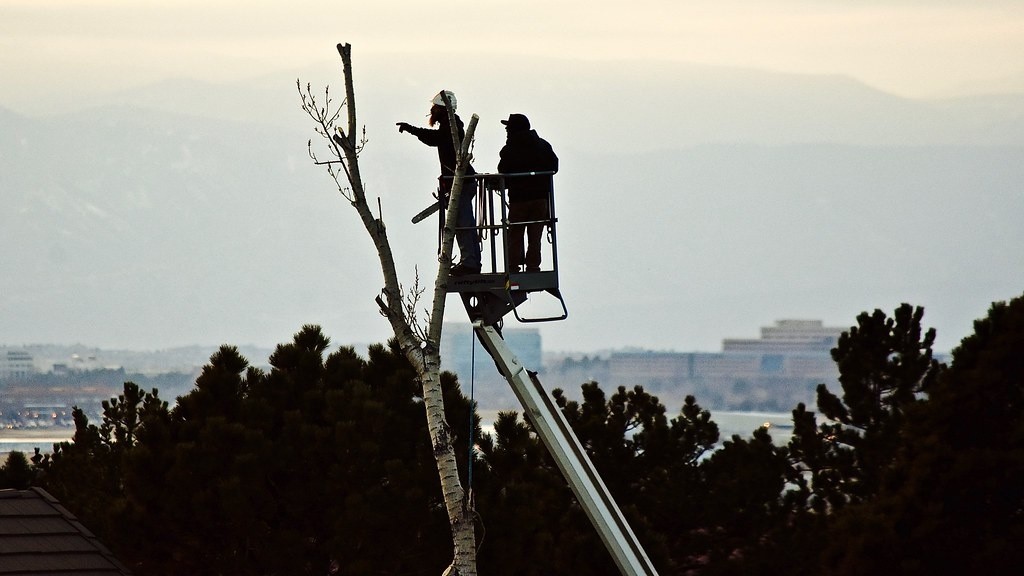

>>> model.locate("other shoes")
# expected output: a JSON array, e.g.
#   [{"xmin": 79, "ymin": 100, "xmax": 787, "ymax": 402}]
[
  {"xmin": 526, "ymin": 267, "xmax": 540, "ymax": 273},
  {"xmin": 449, "ymin": 263, "xmax": 480, "ymax": 277},
  {"xmin": 509, "ymin": 266, "xmax": 520, "ymax": 272}
]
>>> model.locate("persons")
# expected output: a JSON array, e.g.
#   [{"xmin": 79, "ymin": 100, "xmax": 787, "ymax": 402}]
[
  {"xmin": 397, "ymin": 91, "xmax": 483, "ymax": 273},
  {"xmin": 498, "ymin": 113, "xmax": 559, "ymax": 273}
]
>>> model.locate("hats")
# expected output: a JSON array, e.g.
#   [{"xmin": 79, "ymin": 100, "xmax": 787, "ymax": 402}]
[{"xmin": 501, "ymin": 114, "xmax": 531, "ymax": 130}]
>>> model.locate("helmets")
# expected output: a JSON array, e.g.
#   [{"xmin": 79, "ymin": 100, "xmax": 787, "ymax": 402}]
[{"xmin": 431, "ymin": 90, "xmax": 457, "ymax": 109}]
